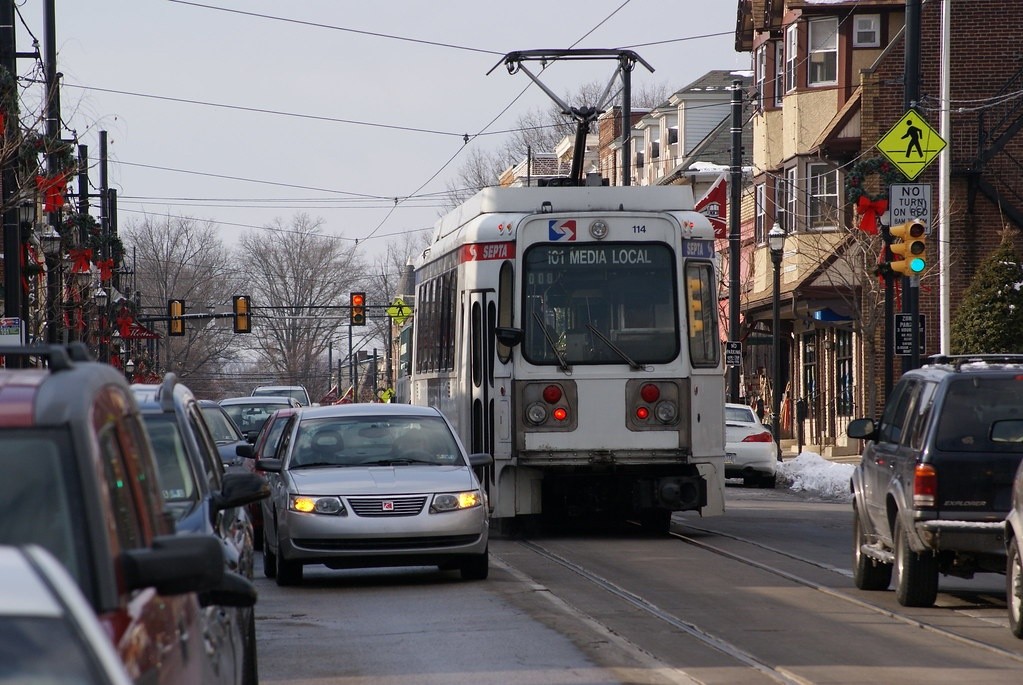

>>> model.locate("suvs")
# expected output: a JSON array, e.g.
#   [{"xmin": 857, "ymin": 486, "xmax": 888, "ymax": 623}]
[
  {"xmin": 0, "ymin": 342, "xmax": 227, "ymax": 685},
  {"xmin": 129, "ymin": 372, "xmax": 273, "ymax": 685},
  {"xmin": 846, "ymin": 354, "xmax": 1023, "ymax": 609}
]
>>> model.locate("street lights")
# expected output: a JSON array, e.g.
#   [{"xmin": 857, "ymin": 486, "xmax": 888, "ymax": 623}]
[
  {"xmin": 40, "ymin": 225, "xmax": 61, "ymax": 343},
  {"xmin": 94, "ymin": 287, "xmax": 108, "ymax": 362},
  {"xmin": 767, "ymin": 222, "xmax": 787, "ymax": 462},
  {"xmin": 126, "ymin": 359, "xmax": 135, "ymax": 381},
  {"xmin": 111, "ymin": 329, "xmax": 121, "ymax": 352}
]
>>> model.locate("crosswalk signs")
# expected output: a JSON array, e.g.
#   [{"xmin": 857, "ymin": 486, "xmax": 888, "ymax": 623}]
[
  {"xmin": 387, "ymin": 298, "xmax": 412, "ymax": 324},
  {"xmin": 381, "ymin": 388, "xmax": 395, "ymax": 403}
]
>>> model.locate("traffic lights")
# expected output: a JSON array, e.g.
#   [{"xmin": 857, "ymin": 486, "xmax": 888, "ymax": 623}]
[
  {"xmin": 890, "ymin": 224, "xmax": 906, "ymax": 276},
  {"xmin": 905, "ymin": 221, "xmax": 926, "ymax": 275},
  {"xmin": 350, "ymin": 292, "xmax": 366, "ymax": 326}
]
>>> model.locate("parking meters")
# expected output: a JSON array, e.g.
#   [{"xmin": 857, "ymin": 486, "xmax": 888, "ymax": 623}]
[
  {"xmin": 756, "ymin": 398, "xmax": 765, "ymax": 424},
  {"xmin": 796, "ymin": 397, "xmax": 805, "ymax": 454}
]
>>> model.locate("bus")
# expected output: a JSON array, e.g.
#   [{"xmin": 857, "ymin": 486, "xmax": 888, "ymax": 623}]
[{"xmin": 410, "ymin": 184, "xmax": 727, "ymax": 542}]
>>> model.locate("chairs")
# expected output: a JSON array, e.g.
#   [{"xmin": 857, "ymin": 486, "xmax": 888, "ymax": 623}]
[
  {"xmin": 309, "ymin": 430, "xmax": 343, "ymax": 464},
  {"xmin": 388, "ymin": 428, "xmax": 436, "ymax": 463}
]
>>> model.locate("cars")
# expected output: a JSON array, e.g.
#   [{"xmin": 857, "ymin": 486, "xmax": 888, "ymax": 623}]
[
  {"xmin": 236, "ymin": 408, "xmax": 395, "ymax": 552},
  {"xmin": 196, "ymin": 399, "xmax": 259, "ymax": 467},
  {"xmin": 251, "ymin": 384, "xmax": 321, "ymax": 407},
  {"xmin": 725, "ymin": 403, "xmax": 777, "ymax": 488},
  {"xmin": 0, "ymin": 544, "xmax": 131, "ymax": 685},
  {"xmin": 990, "ymin": 417, "xmax": 1023, "ymax": 639},
  {"xmin": 217, "ymin": 397, "xmax": 302, "ymax": 442},
  {"xmin": 259, "ymin": 404, "xmax": 495, "ymax": 587}
]
